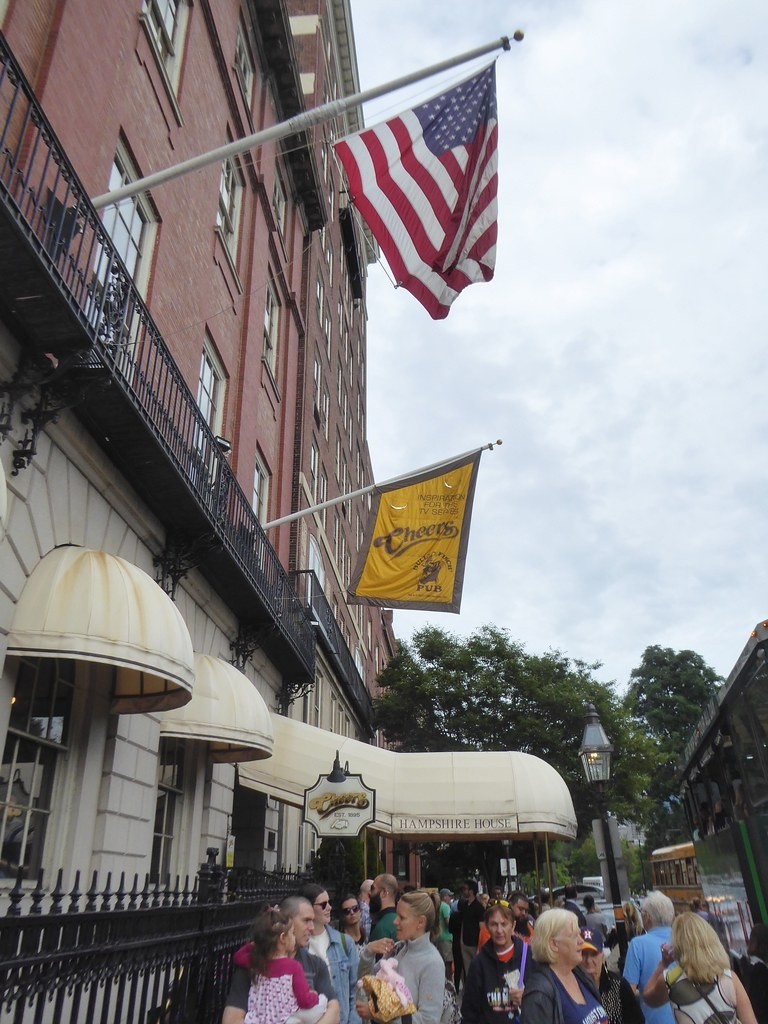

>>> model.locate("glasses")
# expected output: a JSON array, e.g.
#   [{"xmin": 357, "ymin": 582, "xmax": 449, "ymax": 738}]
[
  {"xmin": 463, "ymin": 887, "xmax": 469, "ymax": 891},
  {"xmin": 343, "ymin": 905, "xmax": 359, "ymax": 914},
  {"xmin": 371, "ymin": 886, "xmax": 385, "ymax": 891},
  {"xmin": 313, "ymin": 899, "xmax": 332, "ymax": 909}
]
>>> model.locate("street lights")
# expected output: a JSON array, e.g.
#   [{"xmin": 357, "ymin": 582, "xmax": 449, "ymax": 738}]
[
  {"xmin": 501, "ymin": 839, "xmax": 513, "ymax": 895},
  {"xmin": 577, "ymin": 700, "xmax": 628, "ymax": 976},
  {"xmin": 625, "ymin": 840, "xmax": 647, "ymax": 897}
]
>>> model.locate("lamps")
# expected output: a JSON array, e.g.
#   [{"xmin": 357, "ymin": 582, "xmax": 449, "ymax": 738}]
[{"xmin": 213, "ymin": 436, "xmax": 231, "ymax": 452}]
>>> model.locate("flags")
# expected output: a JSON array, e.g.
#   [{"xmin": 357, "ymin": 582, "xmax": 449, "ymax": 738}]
[
  {"xmin": 332, "ymin": 60, "xmax": 498, "ymax": 320},
  {"xmin": 346, "ymin": 450, "xmax": 481, "ymax": 614}
]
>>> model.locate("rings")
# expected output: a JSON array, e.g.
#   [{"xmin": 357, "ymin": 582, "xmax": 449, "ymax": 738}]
[
  {"xmin": 662, "ymin": 948, "xmax": 663, "ymax": 952},
  {"xmin": 386, "ymin": 947, "xmax": 387, "ymax": 950}
]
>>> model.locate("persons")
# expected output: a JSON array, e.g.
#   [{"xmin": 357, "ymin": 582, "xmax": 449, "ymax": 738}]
[
  {"xmin": 577, "ymin": 927, "xmax": 645, "ymax": 1024},
  {"xmin": 460, "ymin": 904, "xmax": 531, "ymax": 1024},
  {"xmin": 329, "ymin": 873, "xmax": 650, "ymax": 995},
  {"xmin": 521, "ymin": 908, "xmax": 608, "ymax": 1024},
  {"xmin": 688, "ymin": 897, "xmax": 708, "ymax": 924},
  {"xmin": 295, "ymin": 883, "xmax": 362, "ymax": 1024},
  {"xmin": 222, "ymin": 896, "xmax": 340, "ymax": 1024},
  {"xmin": 355, "ymin": 890, "xmax": 445, "ymax": 1024},
  {"xmin": 234, "ymin": 905, "xmax": 320, "ymax": 1024},
  {"xmin": 622, "ymin": 891, "xmax": 680, "ymax": 1024},
  {"xmin": 642, "ymin": 912, "xmax": 758, "ymax": 1024}
]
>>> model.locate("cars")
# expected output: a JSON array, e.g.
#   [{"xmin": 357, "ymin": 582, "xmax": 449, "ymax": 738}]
[{"xmin": 529, "ymin": 885, "xmax": 647, "ymax": 965}]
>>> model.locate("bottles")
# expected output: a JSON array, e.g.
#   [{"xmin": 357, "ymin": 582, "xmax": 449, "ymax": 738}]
[{"xmin": 356, "ymin": 980, "xmax": 372, "ymax": 1024}]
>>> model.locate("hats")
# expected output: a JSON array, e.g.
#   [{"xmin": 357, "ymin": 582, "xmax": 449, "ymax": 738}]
[
  {"xmin": 439, "ymin": 888, "xmax": 454, "ymax": 895},
  {"xmin": 579, "ymin": 927, "xmax": 603, "ymax": 952}
]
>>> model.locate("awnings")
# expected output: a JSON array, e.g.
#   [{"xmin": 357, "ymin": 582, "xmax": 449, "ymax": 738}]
[
  {"xmin": 6, "ymin": 545, "xmax": 193, "ymax": 715},
  {"xmin": 162, "ymin": 653, "xmax": 274, "ymax": 764},
  {"xmin": 237, "ymin": 708, "xmax": 578, "ymax": 874}
]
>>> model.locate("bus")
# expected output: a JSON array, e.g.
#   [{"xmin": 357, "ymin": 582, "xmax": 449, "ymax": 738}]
[
  {"xmin": 583, "ymin": 877, "xmax": 604, "ymax": 888},
  {"xmin": 675, "ymin": 618, "xmax": 768, "ymax": 1024},
  {"xmin": 651, "ymin": 842, "xmax": 708, "ymax": 926}
]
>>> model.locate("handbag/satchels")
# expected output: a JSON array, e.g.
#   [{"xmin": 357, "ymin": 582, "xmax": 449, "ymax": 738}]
[
  {"xmin": 355, "ymin": 975, "xmax": 416, "ymax": 1024},
  {"xmin": 440, "ymin": 980, "xmax": 463, "ymax": 1024}
]
